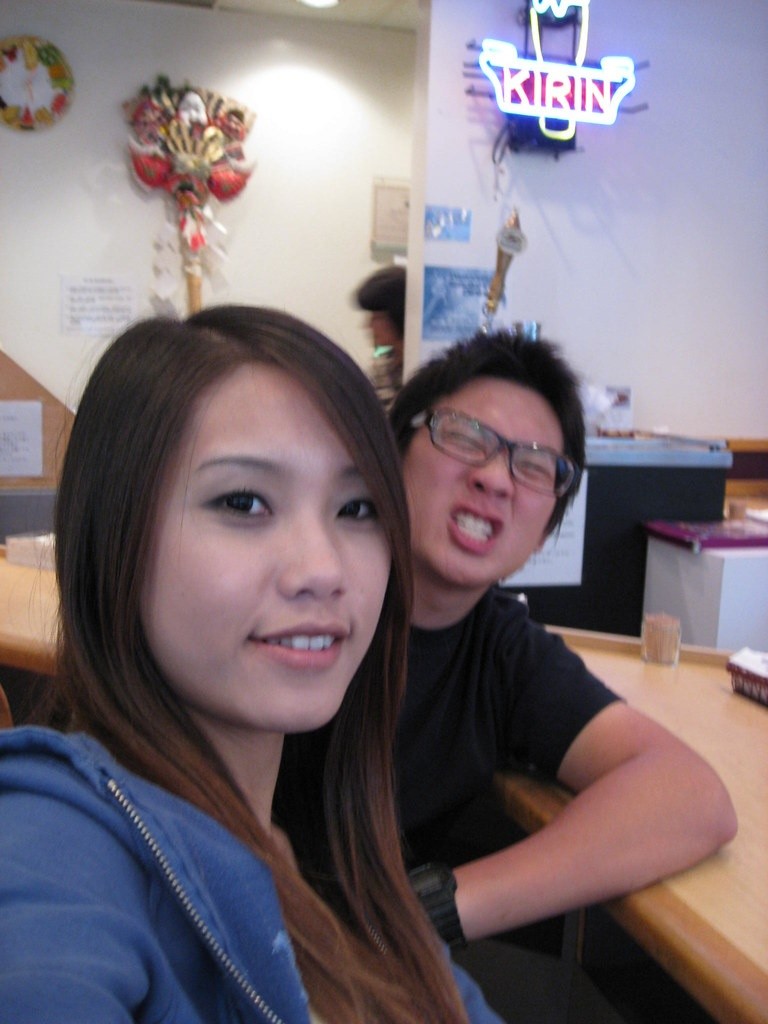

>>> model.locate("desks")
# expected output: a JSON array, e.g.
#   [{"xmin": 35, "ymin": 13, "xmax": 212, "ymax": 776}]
[{"xmin": 0, "ymin": 538, "xmax": 768, "ymax": 1024}]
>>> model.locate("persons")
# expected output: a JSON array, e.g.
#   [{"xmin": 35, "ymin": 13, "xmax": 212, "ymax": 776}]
[
  {"xmin": 0, "ymin": 306, "xmax": 504, "ymax": 1024},
  {"xmin": 379, "ymin": 330, "xmax": 735, "ymax": 1024},
  {"xmin": 355, "ymin": 264, "xmax": 406, "ymax": 408}
]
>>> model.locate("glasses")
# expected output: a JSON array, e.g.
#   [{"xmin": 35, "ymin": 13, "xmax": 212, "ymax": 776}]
[{"xmin": 407, "ymin": 408, "xmax": 581, "ymax": 497}]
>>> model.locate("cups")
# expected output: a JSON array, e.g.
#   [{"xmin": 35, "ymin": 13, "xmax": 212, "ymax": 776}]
[{"xmin": 640, "ymin": 620, "xmax": 684, "ymax": 668}]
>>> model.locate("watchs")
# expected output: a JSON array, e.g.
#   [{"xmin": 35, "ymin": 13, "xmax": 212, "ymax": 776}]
[{"xmin": 408, "ymin": 864, "xmax": 468, "ymax": 947}]
